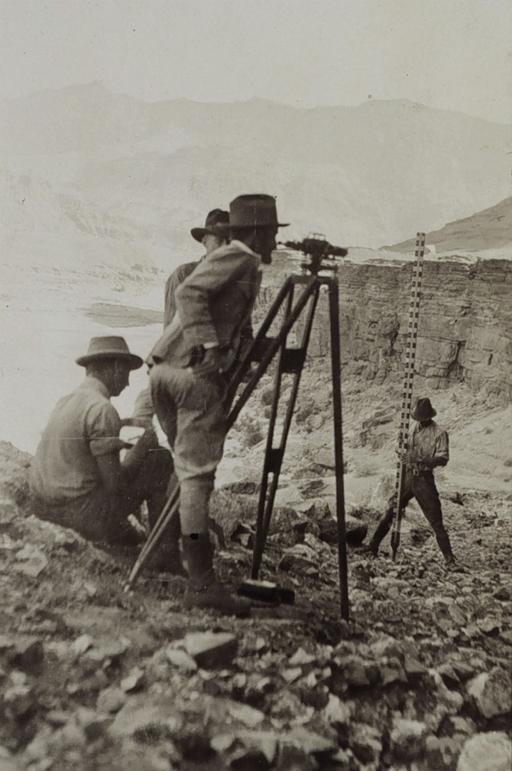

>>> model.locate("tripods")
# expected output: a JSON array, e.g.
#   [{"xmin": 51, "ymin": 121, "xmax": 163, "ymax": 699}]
[{"xmin": 123, "ymin": 275, "xmax": 349, "ymax": 621}]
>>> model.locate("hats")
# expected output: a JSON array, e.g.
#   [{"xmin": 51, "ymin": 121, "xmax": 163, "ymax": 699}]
[
  {"xmin": 76, "ymin": 336, "xmax": 146, "ymax": 371},
  {"xmin": 410, "ymin": 396, "xmax": 437, "ymax": 422},
  {"xmin": 191, "ymin": 193, "xmax": 291, "ymax": 242}
]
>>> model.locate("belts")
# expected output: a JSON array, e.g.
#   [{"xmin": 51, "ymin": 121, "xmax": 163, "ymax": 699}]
[{"xmin": 406, "ymin": 468, "xmax": 433, "ymax": 476}]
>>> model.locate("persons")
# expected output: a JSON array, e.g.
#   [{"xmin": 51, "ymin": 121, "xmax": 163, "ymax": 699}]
[
  {"xmin": 146, "ymin": 193, "xmax": 278, "ymax": 613},
  {"xmin": 31, "ymin": 335, "xmax": 186, "ymax": 578},
  {"xmin": 355, "ymin": 399, "xmax": 463, "ymax": 571},
  {"xmin": 165, "ymin": 209, "xmax": 260, "ymax": 384}
]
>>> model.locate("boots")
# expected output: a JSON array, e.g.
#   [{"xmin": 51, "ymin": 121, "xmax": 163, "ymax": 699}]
[
  {"xmin": 146, "ymin": 511, "xmax": 187, "ymax": 577},
  {"xmin": 180, "ymin": 531, "xmax": 253, "ymax": 615},
  {"xmin": 354, "ymin": 520, "xmax": 392, "ymax": 556},
  {"xmin": 437, "ymin": 534, "xmax": 463, "ymax": 574}
]
